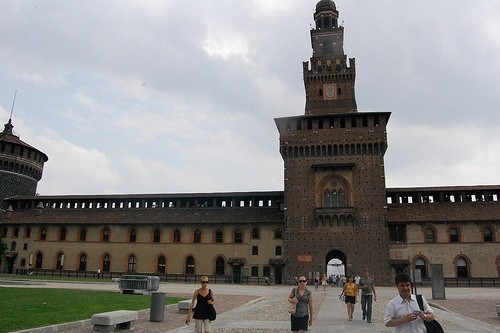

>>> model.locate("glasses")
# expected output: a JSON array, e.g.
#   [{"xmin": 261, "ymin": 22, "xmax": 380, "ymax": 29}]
[
  {"xmin": 298, "ymin": 280, "xmax": 306, "ymax": 283},
  {"xmin": 201, "ymin": 281, "xmax": 209, "ymax": 284}
]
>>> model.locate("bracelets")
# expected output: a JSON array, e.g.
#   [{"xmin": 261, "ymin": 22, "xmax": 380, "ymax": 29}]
[{"xmin": 424, "ymin": 315, "xmax": 426, "ymax": 320}]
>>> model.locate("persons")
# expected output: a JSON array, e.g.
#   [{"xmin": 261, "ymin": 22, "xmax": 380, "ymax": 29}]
[
  {"xmin": 357, "ymin": 270, "xmax": 376, "ymax": 323},
  {"xmin": 97, "ymin": 267, "xmax": 101, "ymax": 279},
  {"xmin": 384, "ymin": 274, "xmax": 434, "ymax": 333},
  {"xmin": 288, "ymin": 275, "xmax": 314, "ymax": 333},
  {"xmin": 314, "ymin": 273, "xmax": 347, "ymax": 291},
  {"xmin": 354, "ymin": 273, "xmax": 361, "ymax": 294},
  {"xmin": 343, "ymin": 276, "xmax": 357, "ymax": 321},
  {"xmin": 186, "ymin": 276, "xmax": 215, "ymax": 333}
]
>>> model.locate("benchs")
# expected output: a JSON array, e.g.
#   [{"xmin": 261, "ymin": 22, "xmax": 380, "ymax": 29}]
[
  {"xmin": 178, "ymin": 298, "xmax": 197, "ymax": 314},
  {"xmin": 91, "ymin": 310, "xmax": 139, "ymax": 333},
  {"xmin": 496, "ymin": 305, "xmax": 500, "ymax": 317}
]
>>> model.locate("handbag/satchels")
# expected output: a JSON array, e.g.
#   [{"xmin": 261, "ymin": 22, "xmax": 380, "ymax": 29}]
[
  {"xmin": 209, "ymin": 304, "xmax": 216, "ymax": 321},
  {"xmin": 338, "ymin": 290, "xmax": 346, "ymax": 303},
  {"xmin": 287, "ymin": 288, "xmax": 297, "ymax": 314},
  {"xmin": 416, "ymin": 294, "xmax": 444, "ymax": 333}
]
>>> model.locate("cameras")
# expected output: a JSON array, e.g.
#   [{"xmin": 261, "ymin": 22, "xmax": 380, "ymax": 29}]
[{"xmin": 413, "ymin": 310, "xmax": 421, "ymax": 316}]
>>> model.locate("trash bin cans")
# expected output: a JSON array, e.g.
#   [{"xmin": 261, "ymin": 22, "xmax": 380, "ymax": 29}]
[{"xmin": 150, "ymin": 293, "xmax": 166, "ymax": 321}]
